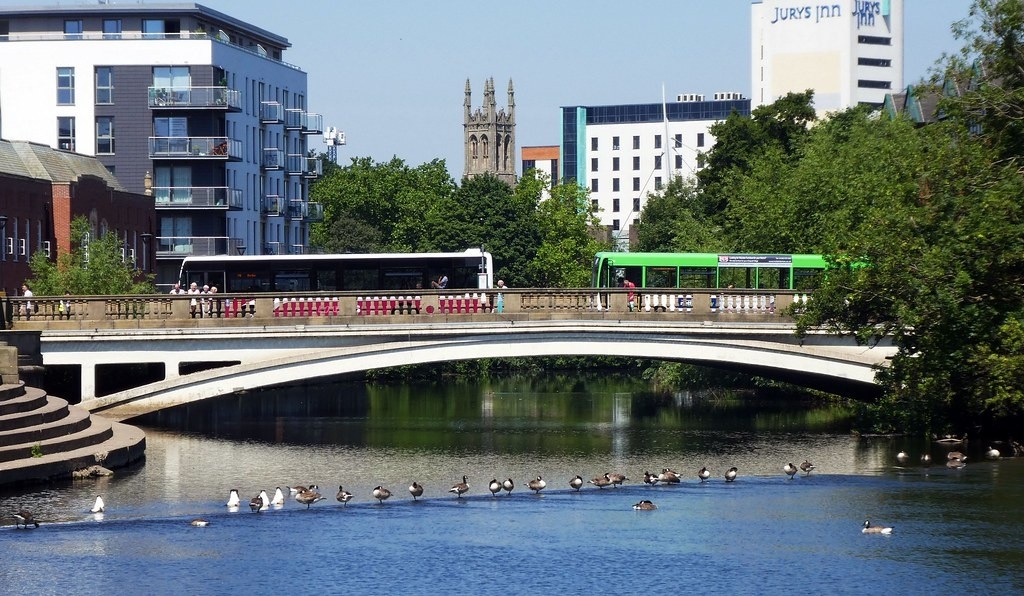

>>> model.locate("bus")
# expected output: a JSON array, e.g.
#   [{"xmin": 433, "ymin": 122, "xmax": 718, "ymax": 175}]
[
  {"xmin": 589, "ymin": 252, "xmax": 877, "ymax": 314},
  {"xmin": 179, "ymin": 247, "xmax": 494, "ymax": 315}
]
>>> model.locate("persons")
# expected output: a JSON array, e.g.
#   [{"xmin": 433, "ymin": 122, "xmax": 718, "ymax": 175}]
[
  {"xmin": 618, "ymin": 278, "xmax": 624, "ymax": 287},
  {"xmin": 208, "ymin": 141, "xmax": 230, "ymax": 155},
  {"xmin": 168, "ymin": 284, "xmax": 186, "ymax": 294},
  {"xmin": 416, "ymin": 284, "xmax": 422, "ymax": 288},
  {"xmin": 60, "ymin": 291, "xmax": 71, "ymax": 320},
  {"xmin": 200, "ymin": 285, "xmax": 217, "ymax": 317},
  {"xmin": 432, "ymin": 273, "xmax": 448, "ymax": 289},
  {"xmin": 497, "ymin": 280, "xmax": 508, "ymax": 313},
  {"xmin": 623, "ymin": 280, "xmax": 635, "ymax": 312},
  {"xmin": 21, "ymin": 284, "xmax": 33, "ymax": 321},
  {"xmin": 188, "ymin": 282, "xmax": 201, "ymax": 317}
]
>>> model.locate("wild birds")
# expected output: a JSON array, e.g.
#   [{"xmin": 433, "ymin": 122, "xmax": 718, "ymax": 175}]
[
  {"xmin": 336, "ymin": 485, "xmax": 354, "ymax": 505},
  {"xmin": 258, "ymin": 490, "xmax": 271, "ymax": 511},
  {"xmin": 698, "ymin": 467, "xmax": 710, "ymax": 483},
  {"xmin": 249, "ymin": 495, "xmax": 263, "ymax": 513},
  {"xmin": 896, "ymin": 447, "xmax": 1001, "ymax": 469},
  {"xmin": 409, "ymin": 481, "xmax": 424, "ymax": 499},
  {"xmin": 799, "ymin": 459, "xmax": 817, "ymax": 475},
  {"xmin": 286, "ymin": 484, "xmax": 319, "ymax": 492},
  {"xmin": 784, "ymin": 462, "xmax": 798, "ymax": 479},
  {"xmin": 295, "ymin": 490, "xmax": 327, "ymax": 509},
  {"xmin": 503, "ymin": 478, "xmax": 514, "ymax": 495},
  {"xmin": 859, "ymin": 520, "xmax": 898, "ymax": 532},
  {"xmin": 568, "ymin": 474, "xmax": 583, "ymax": 492},
  {"xmin": 724, "ymin": 467, "xmax": 738, "ymax": 482},
  {"xmin": 192, "ymin": 519, "xmax": 210, "ymax": 526},
  {"xmin": 448, "ymin": 475, "xmax": 470, "ymax": 497},
  {"xmin": 272, "ymin": 487, "xmax": 285, "ymax": 505},
  {"xmin": 489, "ymin": 479, "xmax": 502, "ymax": 495},
  {"xmin": 10, "ymin": 510, "xmax": 39, "ymax": 531},
  {"xmin": 221, "ymin": 488, "xmax": 240, "ymax": 507},
  {"xmin": 523, "ymin": 476, "xmax": 546, "ymax": 493},
  {"xmin": 587, "ymin": 468, "xmax": 684, "ymax": 489},
  {"xmin": 90, "ymin": 495, "xmax": 105, "ymax": 512},
  {"xmin": 372, "ymin": 485, "xmax": 394, "ymax": 503},
  {"xmin": 632, "ymin": 500, "xmax": 658, "ymax": 510}
]
